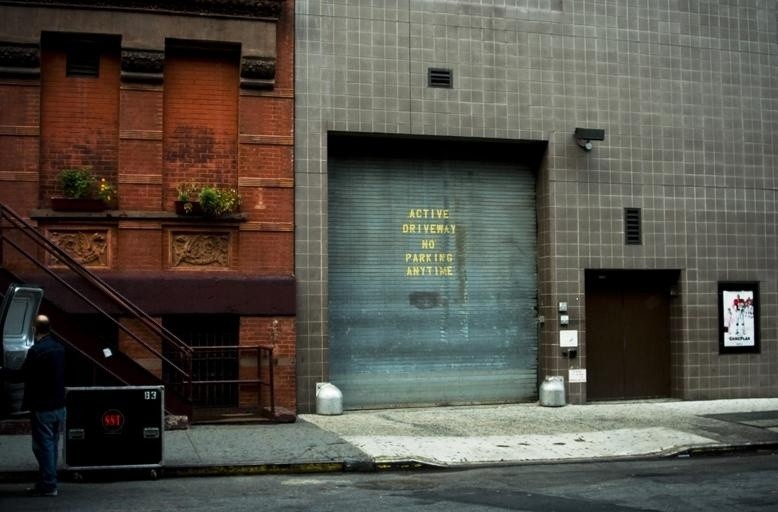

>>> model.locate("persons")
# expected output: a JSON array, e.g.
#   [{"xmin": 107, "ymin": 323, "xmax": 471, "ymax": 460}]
[{"xmin": 0, "ymin": 314, "xmax": 66, "ymax": 496}]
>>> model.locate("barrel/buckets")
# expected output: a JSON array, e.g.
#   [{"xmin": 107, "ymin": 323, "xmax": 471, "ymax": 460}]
[
  {"xmin": 539, "ymin": 374, "xmax": 566, "ymax": 406},
  {"xmin": 316, "ymin": 381, "xmax": 343, "ymax": 415}
]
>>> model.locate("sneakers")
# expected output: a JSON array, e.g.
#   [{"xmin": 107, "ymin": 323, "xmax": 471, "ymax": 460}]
[{"xmin": 24, "ymin": 481, "xmax": 57, "ymax": 496}]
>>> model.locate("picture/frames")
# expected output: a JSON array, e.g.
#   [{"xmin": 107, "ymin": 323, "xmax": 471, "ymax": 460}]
[{"xmin": 717, "ymin": 280, "xmax": 762, "ymax": 355}]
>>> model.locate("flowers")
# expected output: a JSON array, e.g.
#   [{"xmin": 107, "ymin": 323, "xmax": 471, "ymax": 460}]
[
  {"xmin": 60, "ymin": 167, "xmax": 117, "ymax": 202},
  {"xmin": 176, "ymin": 184, "xmax": 242, "ymax": 217}
]
